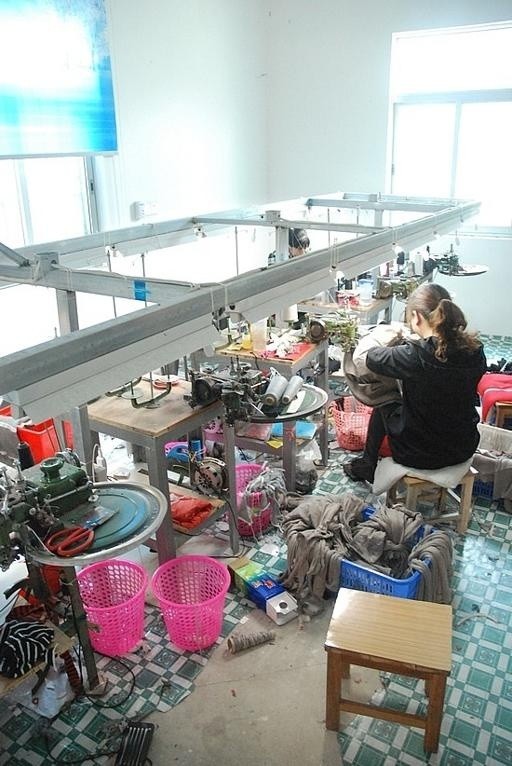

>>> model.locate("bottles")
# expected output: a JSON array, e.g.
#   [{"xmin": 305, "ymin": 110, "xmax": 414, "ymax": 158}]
[{"xmin": 243, "ymin": 330, "xmax": 252, "ymax": 348}]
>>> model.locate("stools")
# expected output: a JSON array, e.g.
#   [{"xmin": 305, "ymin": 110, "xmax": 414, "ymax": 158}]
[
  {"xmin": 492, "ymin": 398, "xmax": 512, "ymax": 429},
  {"xmin": 322, "ymin": 586, "xmax": 455, "ymax": 755},
  {"xmin": 382, "ymin": 467, "xmax": 477, "ymax": 535}
]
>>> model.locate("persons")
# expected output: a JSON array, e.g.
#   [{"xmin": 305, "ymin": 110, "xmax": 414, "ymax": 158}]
[
  {"xmin": 268, "ymin": 228, "xmax": 310, "ymax": 266},
  {"xmin": 347, "ymin": 286, "xmax": 486, "ymax": 483}
]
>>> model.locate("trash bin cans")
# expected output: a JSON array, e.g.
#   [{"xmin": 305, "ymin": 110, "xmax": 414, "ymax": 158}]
[
  {"xmin": 74, "ymin": 558, "xmax": 147, "ymax": 657},
  {"xmin": 233, "ymin": 464, "xmax": 275, "ymax": 537},
  {"xmin": 150, "ymin": 554, "xmax": 232, "ymax": 653},
  {"xmin": 330, "ymin": 395, "xmax": 373, "ymax": 451}
]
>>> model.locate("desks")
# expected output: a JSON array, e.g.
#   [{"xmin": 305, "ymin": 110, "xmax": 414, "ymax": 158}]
[
  {"xmin": 85, "ymin": 373, "xmax": 239, "ymax": 604},
  {"xmin": 193, "ymin": 323, "xmax": 330, "ymax": 495},
  {"xmin": 294, "ymin": 289, "xmax": 395, "ymax": 393}
]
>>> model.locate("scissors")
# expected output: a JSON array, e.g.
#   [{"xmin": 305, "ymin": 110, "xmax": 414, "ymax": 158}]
[
  {"xmin": 45, "ymin": 507, "xmax": 120, "ymax": 556},
  {"xmin": 142, "ymin": 377, "xmax": 179, "ymax": 389}
]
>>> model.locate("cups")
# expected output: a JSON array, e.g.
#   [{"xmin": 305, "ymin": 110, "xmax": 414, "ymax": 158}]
[{"xmin": 358, "ymin": 279, "xmax": 374, "ymax": 305}]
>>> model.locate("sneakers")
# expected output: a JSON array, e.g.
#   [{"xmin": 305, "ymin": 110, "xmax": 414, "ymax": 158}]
[{"xmin": 351, "ymin": 456, "xmax": 375, "ymax": 483}]
